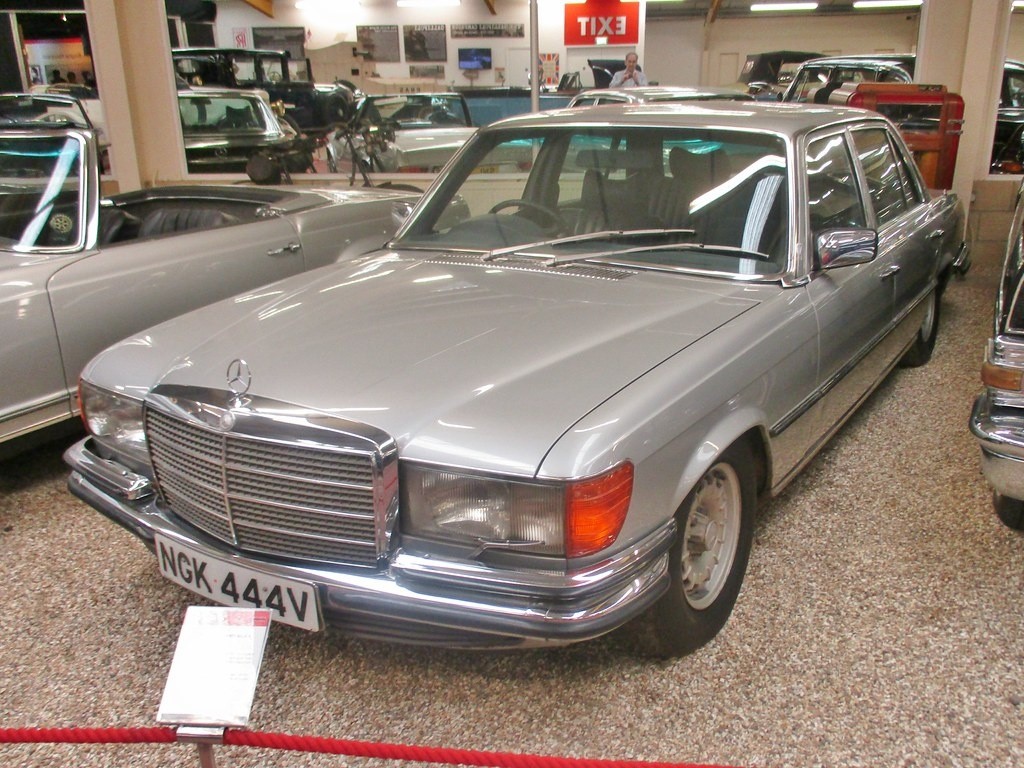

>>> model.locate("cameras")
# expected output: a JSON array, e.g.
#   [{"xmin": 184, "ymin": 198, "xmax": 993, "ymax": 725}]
[{"xmin": 629, "ymin": 73, "xmax": 633, "ymax": 78}]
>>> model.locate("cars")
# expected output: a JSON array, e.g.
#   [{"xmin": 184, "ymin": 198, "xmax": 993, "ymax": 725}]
[
  {"xmin": 60, "ymin": 95, "xmax": 969, "ymax": 660},
  {"xmin": 737, "ymin": 51, "xmax": 833, "ymax": 100},
  {"xmin": 555, "ymin": 85, "xmax": 758, "ymax": 172},
  {"xmin": 1, "ymin": 94, "xmax": 468, "ymax": 446},
  {"xmin": 314, "ymin": 86, "xmax": 534, "ymax": 177},
  {"xmin": 782, "ymin": 52, "xmax": 1024, "ymax": 164},
  {"xmin": 969, "ymin": 170, "xmax": 1024, "ymax": 532},
  {"xmin": 172, "ymin": 48, "xmax": 354, "ymax": 132},
  {"xmin": 0, "ymin": 88, "xmax": 316, "ymax": 172}
]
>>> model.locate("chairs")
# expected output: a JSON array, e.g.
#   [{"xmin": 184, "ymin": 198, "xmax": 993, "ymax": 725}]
[
  {"xmin": 22, "ymin": 203, "xmax": 140, "ymax": 248},
  {"xmin": 141, "ymin": 210, "xmax": 239, "ymax": 239},
  {"xmin": 669, "ymin": 147, "xmax": 731, "ymax": 183},
  {"xmin": 413, "ymin": 102, "xmax": 432, "ymax": 122},
  {"xmin": 587, "ymin": 59, "xmax": 641, "ymax": 88},
  {"xmin": 218, "ymin": 105, "xmax": 258, "ymax": 130},
  {"xmin": 697, "ymin": 172, "xmax": 779, "ymax": 258},
  {"xmin": 575, "ymin": 170, "xmax": 667, "ymax": 244}
]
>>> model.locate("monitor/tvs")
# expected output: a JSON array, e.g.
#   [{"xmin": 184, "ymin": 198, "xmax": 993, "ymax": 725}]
[{"xmin": 458, "ymin": 48, "xmax": 491, "ymax": 69}]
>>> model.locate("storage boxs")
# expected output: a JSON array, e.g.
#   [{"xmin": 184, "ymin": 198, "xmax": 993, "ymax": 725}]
[
  {"xmin": 974, "ymin": 175, "xmax": 1024, "ymax": 211},
  {"xmin": 595, "ymin": 37, "xmax": 607, "ymax": 45},
  {"xmin": 978, "ymin": 212, "xmax": 1015, "ymax": 242}
]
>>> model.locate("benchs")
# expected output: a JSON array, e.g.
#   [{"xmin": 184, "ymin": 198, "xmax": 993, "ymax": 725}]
[{"xmin": 131, "ymin": 197, "xmax": 272, "ymax": 224}]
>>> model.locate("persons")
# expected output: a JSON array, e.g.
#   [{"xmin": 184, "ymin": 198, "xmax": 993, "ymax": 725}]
[
  {"xmin": 67, "ymin": 72, "xmax": 78, "ymax": 83},
  {"xmin": 82, "ymin": 71, "xmax": 97, "ymax": 87},
  {"xmin": 608, "ymin": 52, "xmax": 648, "ymax": 88},
  {"xmin": 51, "ymin": 70, "xmax": 66, "ymax": 83},
  {"xmin": 30, "ymin": 68, "xmax": 42, "ymax": 85}
]
